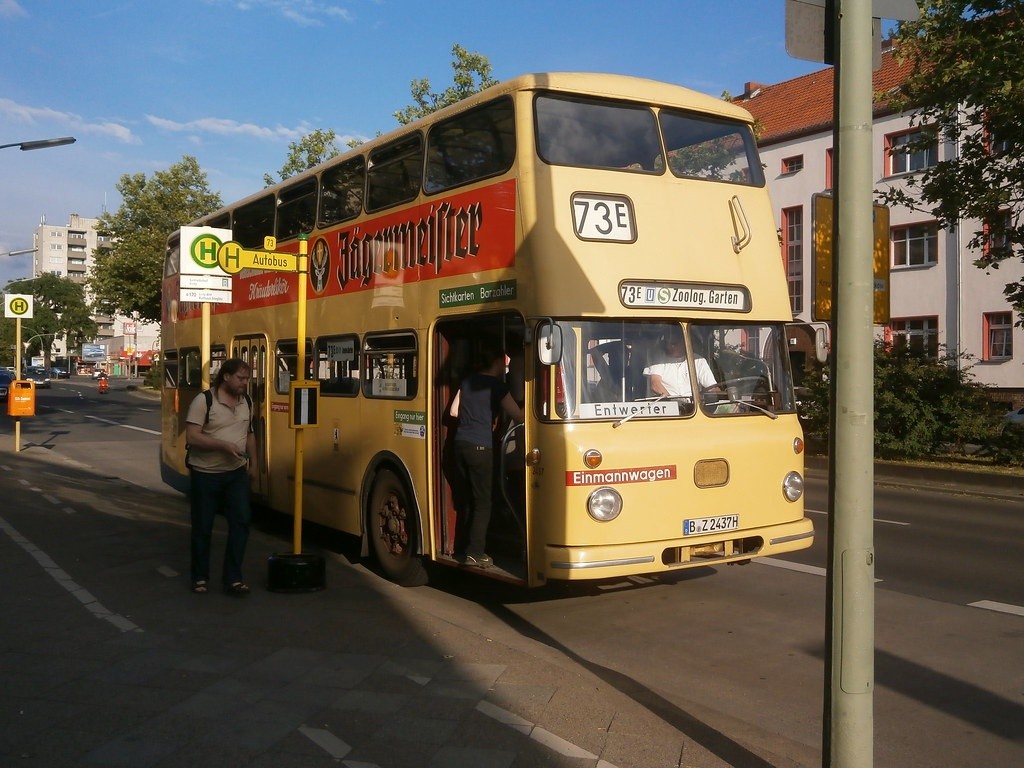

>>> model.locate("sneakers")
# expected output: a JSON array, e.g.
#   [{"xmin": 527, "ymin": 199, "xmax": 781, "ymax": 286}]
[{"xmin": 464, "ymin": 551, "xmax": 493, "ymax": 566}]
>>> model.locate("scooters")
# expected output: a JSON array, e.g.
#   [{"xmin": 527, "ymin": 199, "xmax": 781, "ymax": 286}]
[{"xmin": 98, "ymin": 377, "xmax": 108, "ymax": 393}]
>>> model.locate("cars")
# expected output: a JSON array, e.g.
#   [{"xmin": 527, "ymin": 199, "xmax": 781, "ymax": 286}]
[
  {"xmin": 22, "ymin": 367, "xmax": 51, "ymax": 388},
  {"xmin": 0, "ymin": 370, "xmax": 15, "ymax": 400},
  {"xmin": 92, "ymin": 369, "xmax": 105, "ymax": 378},
  {"xmin": 49, "ymin": 368, "xmax": 70, "ymax": 378}
]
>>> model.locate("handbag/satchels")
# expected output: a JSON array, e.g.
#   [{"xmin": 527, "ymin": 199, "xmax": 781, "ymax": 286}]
[
  {"xmin": 267, "ymin": 554, "xmax": 327, "ymax": 593},
  {"xmin": 448, "ymin": 389, "xmax": 461, "ymax": 418}
]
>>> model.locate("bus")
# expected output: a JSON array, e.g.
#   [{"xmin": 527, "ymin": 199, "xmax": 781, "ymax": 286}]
[{"xmin": 159, "ymin": 71, "xmax": 831, "ymax": 589}]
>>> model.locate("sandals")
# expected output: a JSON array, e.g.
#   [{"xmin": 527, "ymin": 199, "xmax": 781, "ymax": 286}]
[
  {"xmin": 192, "ymin": 580, "xmax": 210, "ymax": 594},
  {"xmin": 229, "ymin": 581, "xmax": 250, "ymax": 594}
]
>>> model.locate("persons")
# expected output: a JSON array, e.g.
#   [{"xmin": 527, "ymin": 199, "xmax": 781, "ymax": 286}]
[
  {"xmin": 98, "ymin": 370, "xmax": 109, "ymax": 386},
  {"xmin": 643, "ymin": 330, "xmax": 721, "ymax": 406},
  {"xmin": 588, "ymin": 335, "xmax": 638, "ymax": 403},
  {"xmin": 443, "ymin": 345, "xmax": 525, "ymax": 567},
  {"xmin": 184, "ymin": 358, "xmax": 259, "ymax": 595}
]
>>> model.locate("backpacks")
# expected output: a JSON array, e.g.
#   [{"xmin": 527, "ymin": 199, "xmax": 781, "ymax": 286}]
[{"xmin": 184, "ymin": 389, "xmax": 253, "ymax": 469}]
